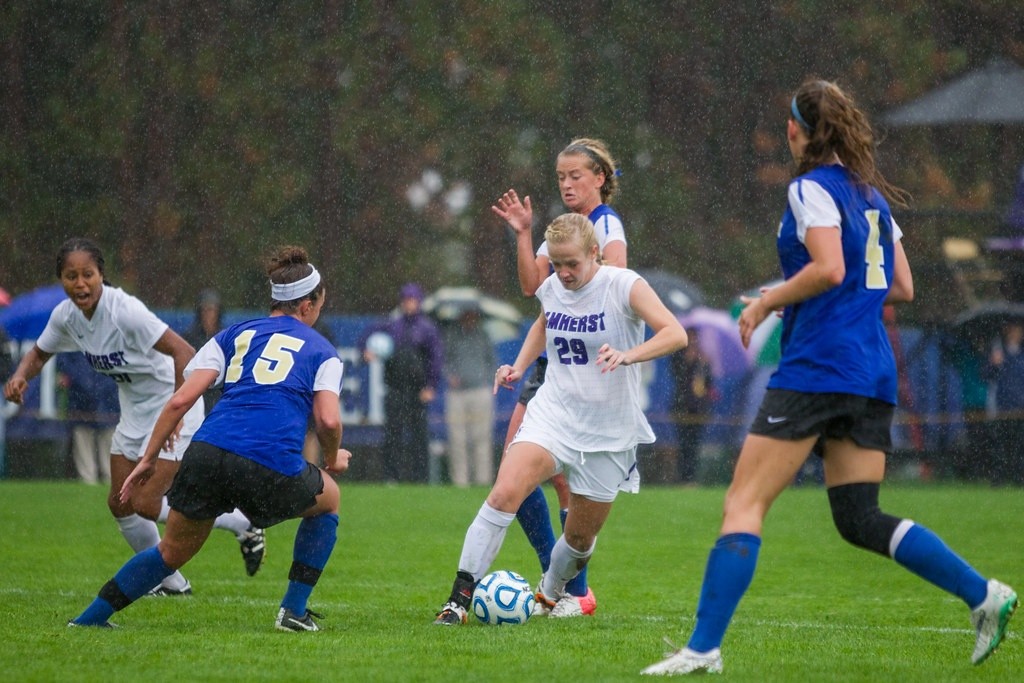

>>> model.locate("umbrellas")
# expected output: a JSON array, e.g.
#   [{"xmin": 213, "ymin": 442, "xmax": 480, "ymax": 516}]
[
  {"xmin": 939, "ymin": 298, "xmax": 1024, "ymax": 354},
  {"xmin": 1, "ymin": 283, "xmax": 70, "ymax": 341},
  {"xmin": 388, "ymin": 268, "xmax": 786, "ymax": 378}
]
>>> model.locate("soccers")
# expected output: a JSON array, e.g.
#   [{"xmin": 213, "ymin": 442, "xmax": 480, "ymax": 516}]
[{"xmin": 472, "ymin": 570, "xmax": 536, "ymax": 627}]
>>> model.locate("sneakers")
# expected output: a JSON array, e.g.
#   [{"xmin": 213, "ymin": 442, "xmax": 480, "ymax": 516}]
[
  {"xmin": 640, "ymin": 638, "xmax": 724, "ymax": 676},
  {"xmin": 275, "ymin": 605, "xmax": 325, "ymax": 634},
  {"xmin": 67, "ymin": 618, "xmax": 121, "ymax": 630},
  {"xmin": 235, "ymin": 523, "xmax": 266, "ymax": 577},
  {"xmin": 532, "ymin": 574, "xmax": 565, "ymax": 617},
  {"xmin": 551, "ymin": 587, "xmax": 597, "ymax": 618},
  {"xmin": 971, "ymin": 578, "xmax": 1020, "ymax": 667},
  {"xmin": 145, "ymin": 580, "xmax": 193, "ymax": 598},
  {"xmin": 434, "ymin": 599, "xmax": 468, "ymax": 626}
]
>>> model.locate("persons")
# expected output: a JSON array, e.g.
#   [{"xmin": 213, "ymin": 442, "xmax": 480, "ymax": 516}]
[
  {"xmin": 55, "ymin": 351, "xmax": 121, "ymax": 486},
  {"xmin": 66, "ymin": 252, "xmax": 352, "ymax": 634},
  {"xmin": 440, "ymin": 305, "xmax": 497, "ymax": 487},
  {"xmin": 988, "ymin": 322, "xmax": 1024, "ymax": 495},
  {"xmin": 666, "ymin": 329, "xmax": 719, "ymax": 489},
  {"xmin": 5, "ymin": 237, "xmax": 268, "ymax": 595},
  {"xmin": 360, "ymin": 284, "xmax": 443, "ymax": 485},
  {"xmin": 639, "ymin": 82, "xmax": 1018, "ymax": 677},
  {"xmin": 489, "ymin": 138, "xmax": 627, "ymax": 620},
  {"xmin": 436, "ymin": 211, "xmax": 688, "ymax": 624},
  {"xmin": 183, "ymin": 287, "xmax": 232, "ymax": 413}
]
